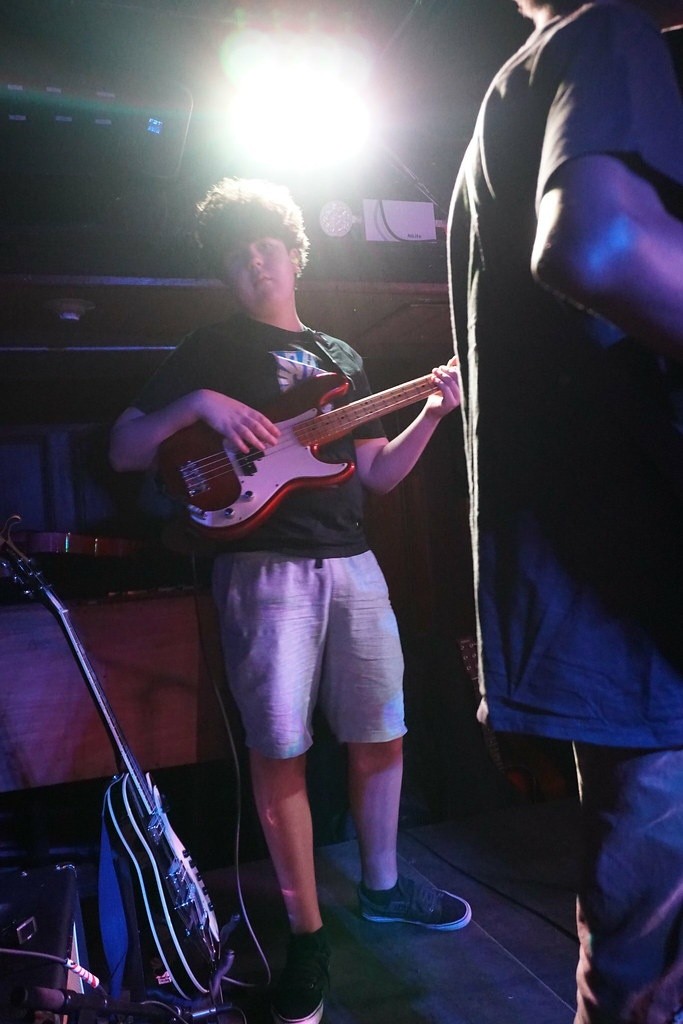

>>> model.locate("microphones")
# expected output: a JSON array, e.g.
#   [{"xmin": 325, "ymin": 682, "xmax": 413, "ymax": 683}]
[{"xmin": 171, "ymin": 1004, "xmax": 232, "ymax": 1024}]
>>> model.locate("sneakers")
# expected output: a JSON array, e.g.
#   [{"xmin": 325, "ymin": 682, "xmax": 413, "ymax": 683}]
[
  {"xmin": 271, "ymin": 924, "xmax": 332, "ymax": 1024},
  {"xmin": 358, "ymin": 876, "xmax": 472, "ymax": 931}
]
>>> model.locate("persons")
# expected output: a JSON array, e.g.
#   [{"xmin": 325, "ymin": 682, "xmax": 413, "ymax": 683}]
[
  {"xmin": 104, "ymin": 176, "xmax": 471, "ymax": 1024},
  {"xmin": 448, "ymin": 1, "xmax": 683, "ymax": 1024}
]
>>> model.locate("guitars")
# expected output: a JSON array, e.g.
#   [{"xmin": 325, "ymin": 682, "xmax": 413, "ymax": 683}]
[
  {"xmin": 0, "ymin": 511, "xmax": 225, "ymax": 1002},
  {"xmin": 157, "ymin": 370, "xmax": 443, "ymax": 543},
  {"xmin": 456, "ymin": 636, "xmax": 538, "ymax": 812}
]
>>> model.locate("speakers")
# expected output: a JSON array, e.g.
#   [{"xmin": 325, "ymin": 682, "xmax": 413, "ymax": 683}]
[
  {"xmin": 0, "ymin": 587, "xmax": 199, "ymax": 793},
  {"xmin": 0, "ymin": 863, "xmax": 90, "ymax": 1024}
]
